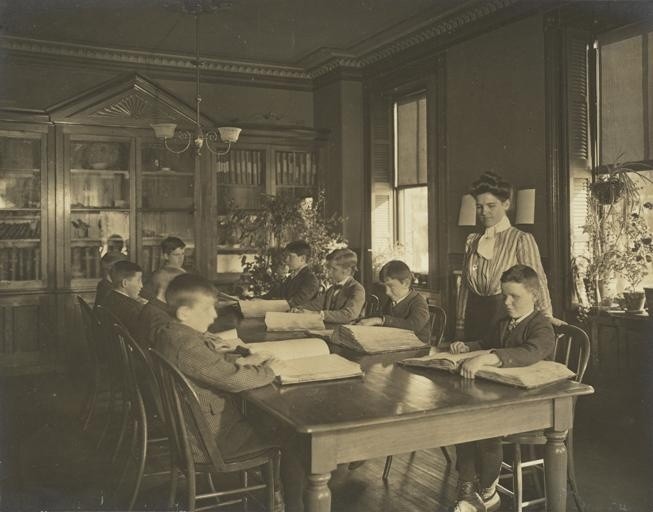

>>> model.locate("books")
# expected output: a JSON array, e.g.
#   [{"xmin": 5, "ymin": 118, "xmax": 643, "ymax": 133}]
[
  {"xmin": 264, "ymin": 306, "xmax": 327, "ymax": 333},
  {"xmin": 0, "ymin": 218, "xmax": 41, "ymax": 282},
  {"xmin": 237, "ymin": 337, "xmax": 366, "ymax": 386},
  {"xmin": 308, "ymin": 322, "xmax": 429, "ymax": 354},
  {"xmin": 218, "ymin": 292, "xmax": 293, "ymax": 317},
  {"xmin": 217, "ymin": 151, "xmax": 313, "ymax": 186},
  {"xmin": 395, "ymin": 347, "xmax": 578, "ymax": 389},
  {"xmin": 206, "ymin": 328, "xmax": 245, "ymax": 354}
]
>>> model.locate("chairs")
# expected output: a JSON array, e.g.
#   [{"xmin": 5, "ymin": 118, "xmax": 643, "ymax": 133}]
[
  {"xmin": 381, "ymin": 305, "xmax": 452, "ymax": 482},
  {"xmin": 495, "ymin": 323, "xmax": 591, "ymax": 510},
  {"xmin": 354, "ymin": 293, "xmax": 380, "ymax": 326},
  {"xmin": 70, "ymin": 288, "xmax": 164, "ymax": 510},
  {"xmin": 144, "ymin": 343, "xmax": 287, "ymax": 512}
]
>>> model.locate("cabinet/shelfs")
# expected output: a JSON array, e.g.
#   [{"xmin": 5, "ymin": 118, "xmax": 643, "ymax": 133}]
[
  {"xmin": 0, "ymin": 108, "xmax": 51, "ymax": 294},
  {"xmin": 562, "ymin": 296, "xmax": 653, "ymax": 414},
  {"xmin": 207, "ymin": 127, "xmax": 328, "ymax": 285},
  {"xmin": 1, "ymin": 290, "xmax": 56, "ymax": 381},
  {"xmin": 47, "ymin": 70, "xmax": 206, "ymax": 292}
]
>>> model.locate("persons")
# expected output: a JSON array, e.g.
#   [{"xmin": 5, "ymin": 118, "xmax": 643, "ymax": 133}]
[
  {"xmin": 455, "ymin": 171, "xmax": 567, "ymax": 343},
  {"xmin": 93, "ymin": 251, "xmax": 129, "ymax": 309},
  {"xmin": 155, "ymin": 274, "xmax": 307, "ymax": 512},
  {"xmin": 244, "ymin": 239, "xmax": 320, "ymax": 308},
  {"xmin": 450, "ymin": 264, "xmax": 555, "ymax": 512},
  {"xmin": 348, "ymin": 261, "xmax": 431, "ymax": 471},
  {"xmin": 291, "ymin": 247, "xmax": 365, "ymax": 326},
  {"xmin": 142, "ymin": 235, "xmax": 188, "ymax": 290},
  {"xmin": 100, "ymin": 261, "xmax": 172, "ymax": 416}
]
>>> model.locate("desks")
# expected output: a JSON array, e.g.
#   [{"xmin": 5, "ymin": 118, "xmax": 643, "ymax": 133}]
[{"xmin": 113, "ymin": 287, "xmax": 596, "ymax": 512}]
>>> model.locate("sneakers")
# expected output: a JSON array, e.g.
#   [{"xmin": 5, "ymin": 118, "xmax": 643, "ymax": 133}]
[{"xmin": 452, "ymin": 481, "xmax": 502, "ymax": 512}]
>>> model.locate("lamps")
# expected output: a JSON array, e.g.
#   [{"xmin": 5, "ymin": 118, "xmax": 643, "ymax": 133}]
[
  {"xmin": 457, "ymin": 195, "xmax": 479, "ymax": 226},
  {"xmin": 150, "ymin": 1, "xmax": 244, "ymax": 157},
  {"xmin": 515, "ymin": 187, "xmax": 534, "ymax": 226}
]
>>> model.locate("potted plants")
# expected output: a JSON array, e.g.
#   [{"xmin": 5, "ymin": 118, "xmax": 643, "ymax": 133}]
[{"xmin": 575, "ymin": 145, "xmax": 653, "ymax": 324}]
